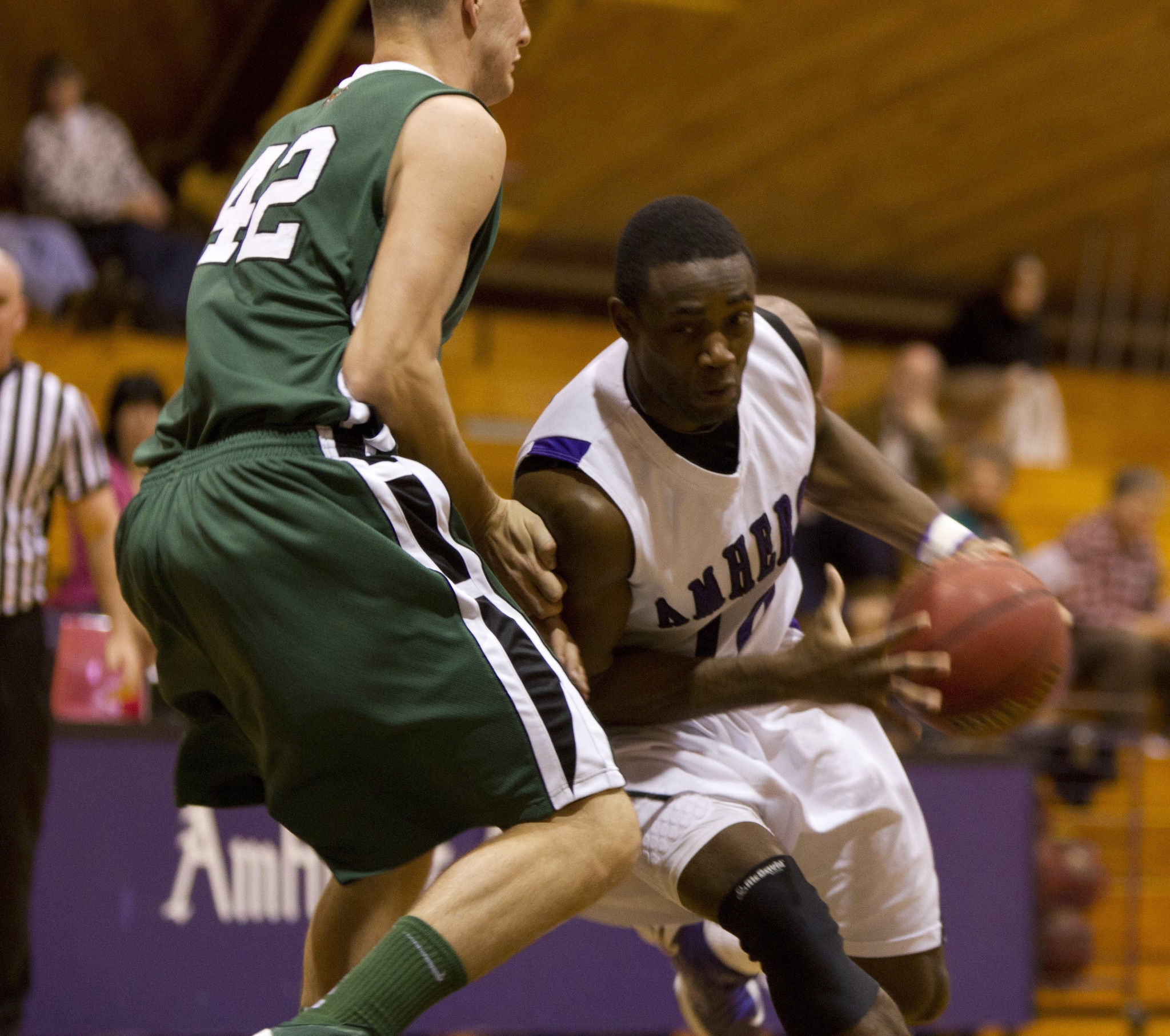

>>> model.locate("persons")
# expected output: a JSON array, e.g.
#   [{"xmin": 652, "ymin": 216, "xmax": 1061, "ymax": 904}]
[
  {"xmin": 113, "ymin": 2, "xmax": 642, "ymax": 1034},
  {"xmin": 513, "ymin": 195, "xmax": 1078, "ymax": 1036},
  {"xmin": 0, "ymin": 246, "xmax": 153, "ymax": 1035},
  {"xmin": 801, "ymin": 249, "xmax": 1169, "ymax": 716},
  {"xmin": 17, "ymin": 46, "xmax": 195, "ymax": 335},
  {"xmin": 54, "ymin": 365, "xmax": 166, "ymax": 626}
]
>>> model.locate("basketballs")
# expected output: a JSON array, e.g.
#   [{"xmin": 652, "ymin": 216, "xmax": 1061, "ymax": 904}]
[{"xmin": 888, "ymin": 555, "xmax": 1066, "ymax": 738}]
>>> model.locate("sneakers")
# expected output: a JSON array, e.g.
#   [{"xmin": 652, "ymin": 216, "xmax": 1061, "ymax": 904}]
[{"xmin": 666, "ymin": 922, "xmax": 765, "ymax": 1036}]
